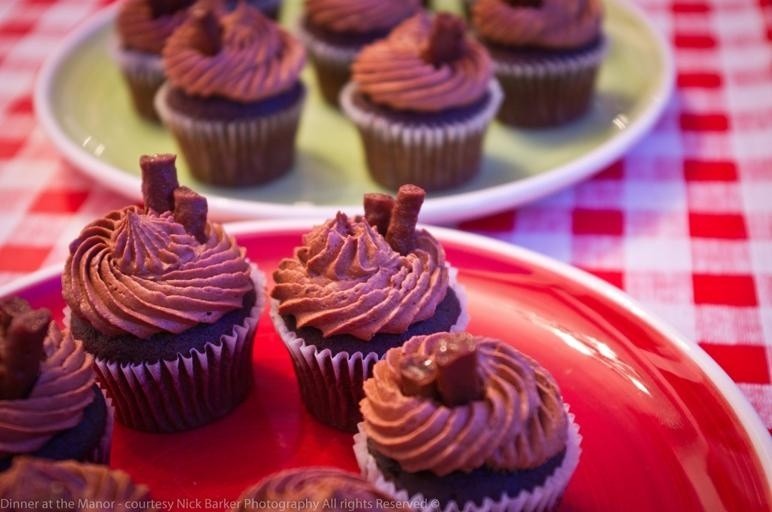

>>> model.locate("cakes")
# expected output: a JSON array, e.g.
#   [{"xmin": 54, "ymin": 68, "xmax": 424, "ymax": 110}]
[
  {"xmin": 62, "ymin": 155, "xmax": 256, "ymax": 432},
  {"xmin": 348, "ymin": 8, "xmax": 494, "ymax": 192},
  {"xmin": 269, "ymin": 183, "xmax": 461, "ymax": 432},
  {"xmin": 231, "ymin": 467, "xmax": 411, "ymax": 511},
  {"xmin": 299, "ymin": 0, "xmax": 433, "ymax": 108},
  {"xmin": 464, "ymin": 0, "xmax": 605, "ymax": 126},
  {"xmin": 0, "ymin": 296, "xmax": 107, "ymax": 473},
  {"xmin": 0, "ymin": 456, "xmax": 154, "ymax": 511},
  {"xmin": 359, "ymin": 331, "xmax": 568, "ymax": 512},
  {"xmin": 113, "ymin": 0, "xmax": 280, "ymax": 122},
  {"xmin": 162, "ymin": 0, "xmax": 307, "ymax": 185}
]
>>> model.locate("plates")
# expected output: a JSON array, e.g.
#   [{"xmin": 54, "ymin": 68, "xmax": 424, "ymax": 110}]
[
  {"xmin": 1, "ymin": 218, "xmax": 772, "ymax": 511},
  {"xmin": 35, "ymin": 0, "xmax": 677, "ymax": 218}
]
